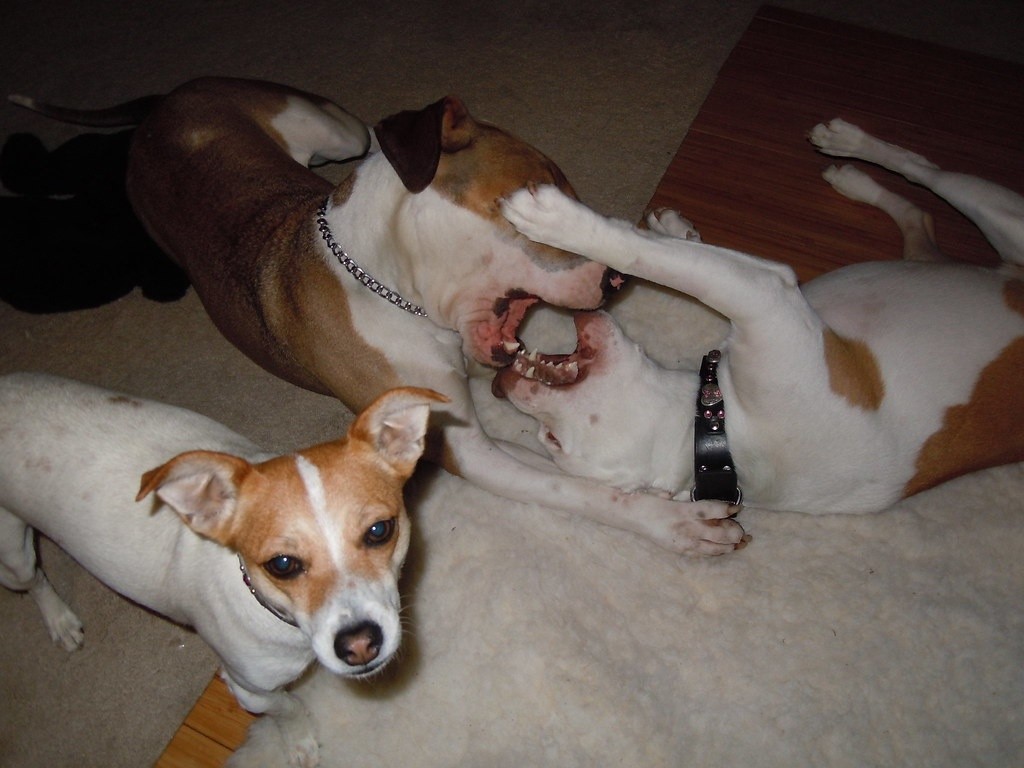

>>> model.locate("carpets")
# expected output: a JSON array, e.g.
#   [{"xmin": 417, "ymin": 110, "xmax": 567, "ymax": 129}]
[
  {"xmin": 227, "ymin": 259, "xmax": 1024, "ymax": 768},
  {"xmin": 0, "ymin": 0, "xmax": 762, "ymax": 768}
]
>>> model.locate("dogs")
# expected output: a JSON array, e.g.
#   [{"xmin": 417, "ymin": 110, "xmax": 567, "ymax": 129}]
[
  {"xmin": 0, "ymin": 369, "xmax": 455, "ymax": 768},
  {"xmin": 7, "ymin": 73, "xmax": 752, "ymax": 562},
  {"xmin": 486, "ymin": 115, "xmax": 1024, "ymax": 518}
]
